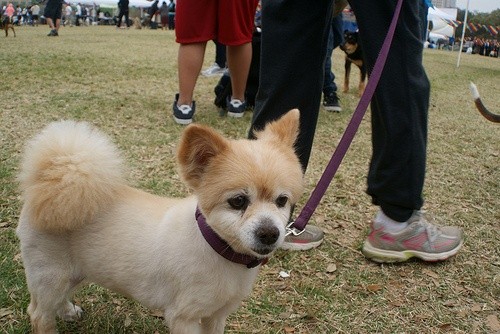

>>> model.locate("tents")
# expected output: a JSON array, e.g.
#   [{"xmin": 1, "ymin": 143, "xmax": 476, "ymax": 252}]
[{"xmin": 426, "ymin": 6, "xmax": 458, "ymax": 43}]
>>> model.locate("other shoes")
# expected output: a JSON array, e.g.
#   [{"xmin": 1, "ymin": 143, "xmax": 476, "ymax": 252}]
[{"xmin": 47, "ymin": 29, "xmax": 58, "ymax": 36}]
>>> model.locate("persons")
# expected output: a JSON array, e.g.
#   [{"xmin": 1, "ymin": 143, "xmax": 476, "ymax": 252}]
[
  {"xmin": 454, "ymin": 37, "xmax": 500, "ymax": 58},
  {"xmin": 173, "ymin": 0, "xmax": 257, "ymax": 126},
  {"xmin": 201, "ymin": 0, "xmax": 343, "ymax": 112},
  {"xmin": 246, "ymin": 0, "xmax": 465, "ymax": 264},
  {"xmin": 0, "ymin": 0, "xmax": 175, "ymax": 37}
]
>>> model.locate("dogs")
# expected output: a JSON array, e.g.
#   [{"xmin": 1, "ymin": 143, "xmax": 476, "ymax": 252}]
[
  {"xmin": 340, "ymin": 29, "xmax": 367, "ymax": 98},
  {"xmin": 16, "ymin": 109, "xmax": 305, "ymax": 334}
]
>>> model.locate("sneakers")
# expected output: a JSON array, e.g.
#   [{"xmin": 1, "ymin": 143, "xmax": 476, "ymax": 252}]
[
  {"xmin": 202, "ymin": 63, "xmax": 229, "ymax": 78},
  {"xmin": 321, "ymin": 93, "xmax": 342, "ymax": 111},
  {"xmin": 281, "ymin": 222, "xmax": 324, "ymax": 251},
  {"xmin": 362, "ymin": 212, "xmax": 464, "ymax": 263},
  {"xmin": 172, "ymin": 93, "xmax": 196, "ymax": 124},
  {"xmin": 227, "ymin": 96, "xmax": 245, "ymax": 118}
]
